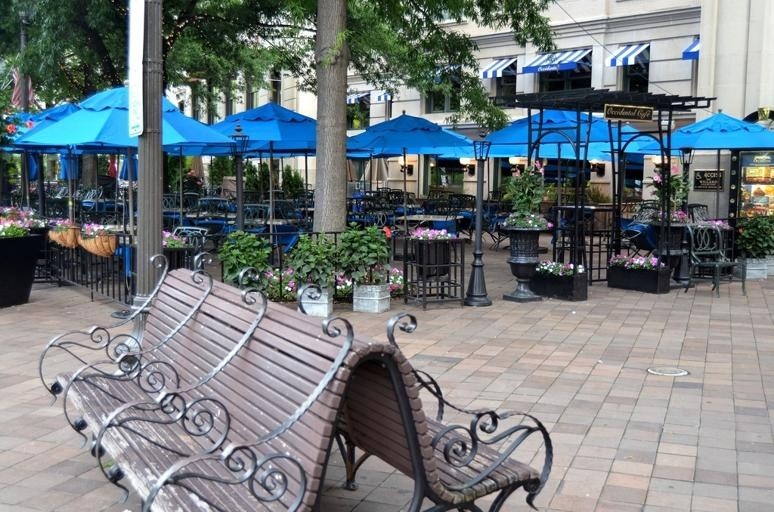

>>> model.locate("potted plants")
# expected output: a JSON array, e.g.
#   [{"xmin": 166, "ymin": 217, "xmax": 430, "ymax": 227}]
[
  {"xmin": 764, "ymin": 215, "xmax": 774, "ymax": 274},
  {"xmin": 287, "ymin": 228, "xmax": 339, "ymax": 318},
  {"xmin": 734, "ymin": 214, "xmax": 770, "ymax": 278},
  {"xmin": 340, "ymin": 225, "xmax": 393, "ymax": 315}
]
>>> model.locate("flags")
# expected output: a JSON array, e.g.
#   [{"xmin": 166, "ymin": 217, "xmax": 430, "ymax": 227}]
[{"xmin": 10, "ymin": 55, "xmax": 33, "ymax": 108}]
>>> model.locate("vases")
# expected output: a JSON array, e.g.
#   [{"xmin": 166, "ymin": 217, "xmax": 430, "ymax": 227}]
[
  {"xmin": 0, "ymin": 235, "xmax": 43, "ymax": 308},
  {"xmin": 30, "ymin": 227, "xmax": 51, "ymax": 246}
]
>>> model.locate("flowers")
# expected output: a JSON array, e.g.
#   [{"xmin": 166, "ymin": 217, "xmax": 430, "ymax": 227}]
[
  {"xmin": 0, "ymin": 218, "xmax": 32, "ymax": 238},
  {"xmin": 51, "ymin": 216, "xmax": 73, "ymax": 228},
  {"xmin": 0, "ymin": 205, "xmax": 51, "ymax": 230}
]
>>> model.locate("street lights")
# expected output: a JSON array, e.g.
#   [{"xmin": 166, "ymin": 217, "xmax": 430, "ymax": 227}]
[
  {"xmin": 673, "ymin": 147, "xmax": 696, "ymax": 283},
  {"xmin": 228, "ymin": 124, "xmax": 250, "ymax": 233},
  {"xmin": 464, "ymin": 129, "xmax": 498, "ymax": 305}
]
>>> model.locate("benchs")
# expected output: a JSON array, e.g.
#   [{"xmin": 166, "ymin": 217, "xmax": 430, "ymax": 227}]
[{"xmin": 39, "ymin": 252, "xmax": 554, "ymax": 512}]
